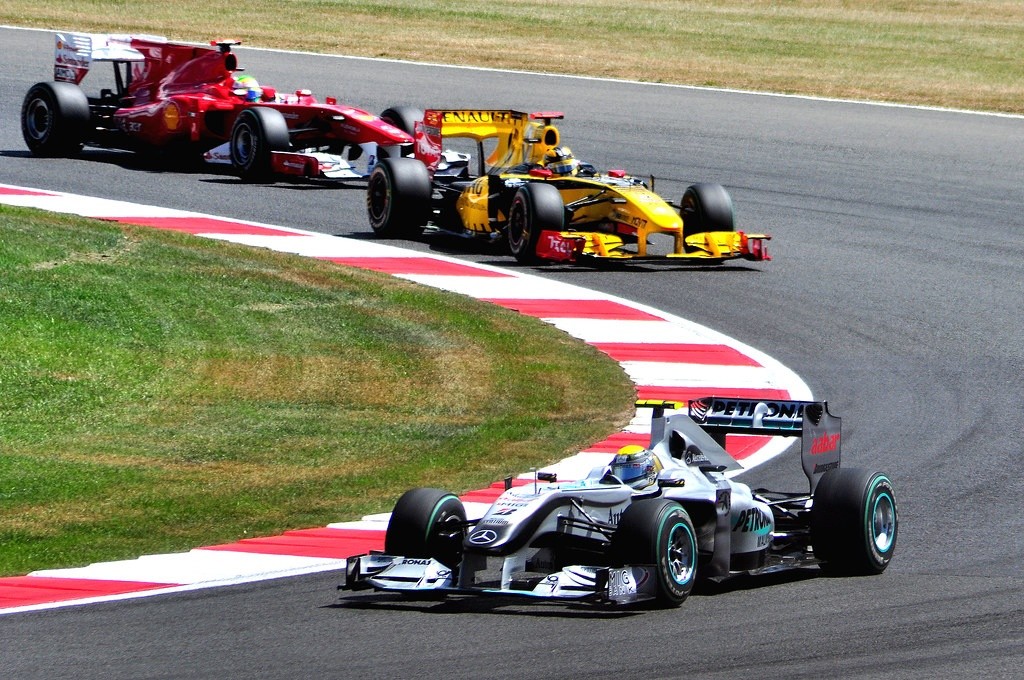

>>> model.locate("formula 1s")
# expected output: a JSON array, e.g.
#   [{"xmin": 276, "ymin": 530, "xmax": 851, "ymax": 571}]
[
  {"xmin": 364, "ymin": 104, "xmax": 774, "ymax": 271},
  {"xmin": 21, "ymin": 31, "xmax": 445, "ymax": 186},
  {"xmin": 336, "ymin": 394, "xmax": 900, "ymax": 614}
]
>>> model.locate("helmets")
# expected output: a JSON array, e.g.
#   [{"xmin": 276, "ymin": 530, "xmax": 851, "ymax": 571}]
[
  {"xmin": 544, "ymin": 145, "xmax": 576, "ymax": 173},
  {"xmin": 608, "ymin": 444, "xmax": 663, "ymax": 491},
  {"xmin": 232, "ymin": 73, "xmax": 263, "ymax": 101}
]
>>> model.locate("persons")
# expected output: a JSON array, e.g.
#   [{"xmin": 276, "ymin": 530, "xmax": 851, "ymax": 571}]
[
  {"xmin": 543, "ymin": 145, "xmax": 578, "ymax": 177},
  {"xmin": 232, "ymin": 74, "xmax": 264, "ymax": 103},
  {"xmin": 608, "ymin": 445, "xmax": 659, "ymax": 492}
]
